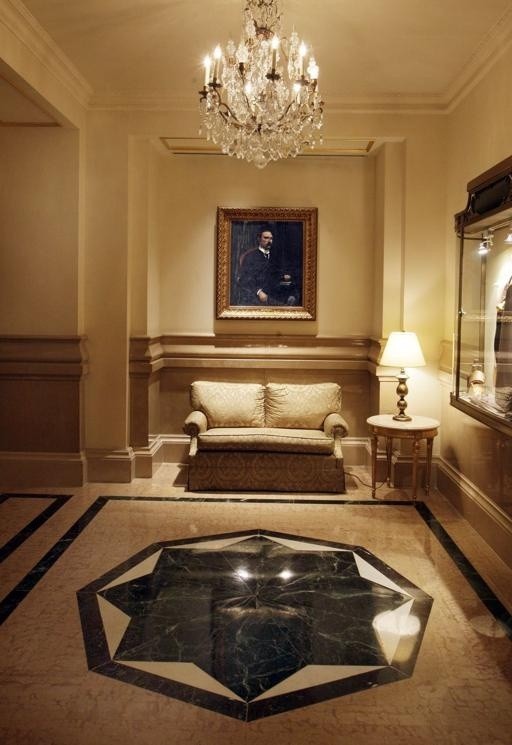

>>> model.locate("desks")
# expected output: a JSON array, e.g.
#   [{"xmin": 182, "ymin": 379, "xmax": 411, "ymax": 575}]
[{"xmin": 367, "ymin": 413, "xmax": 441, "ymax": 507}]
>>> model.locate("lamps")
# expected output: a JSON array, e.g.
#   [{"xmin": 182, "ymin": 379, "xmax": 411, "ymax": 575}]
[
  {"xmin": 477, "ymin": 228, "xmax": 494, "ymax": 255},
  {"xmin": 378, "ymin": 330, "xmax": 427, "ymax": 422},
  {"xmin": 197, "ymin": 0, "xmax": 326, "ymax": 168}
]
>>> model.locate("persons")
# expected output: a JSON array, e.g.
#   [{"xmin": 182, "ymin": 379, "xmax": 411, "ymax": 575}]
[{"xmin": 236, "ymin": 228, "xmax": 291, "ymax": 305}]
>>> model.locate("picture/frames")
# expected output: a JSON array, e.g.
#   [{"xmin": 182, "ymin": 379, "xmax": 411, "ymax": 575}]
[{"xmin": 212, "ymin": 205, "xmax": 318, "ymax": 323}]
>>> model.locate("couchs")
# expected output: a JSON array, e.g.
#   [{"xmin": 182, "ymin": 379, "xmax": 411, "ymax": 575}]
[{"xmin": 182, "ymin": 380, "xmax": 352, "ymax": 494}]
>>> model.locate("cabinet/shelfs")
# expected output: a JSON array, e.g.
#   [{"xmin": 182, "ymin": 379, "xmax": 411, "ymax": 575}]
[{"xmin": 448, "ymin": 154, "xmax": 512, "ymax": 439}]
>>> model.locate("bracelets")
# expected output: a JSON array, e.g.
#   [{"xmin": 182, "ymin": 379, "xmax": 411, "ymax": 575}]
[{"xmin": 256, "ymin": 288, "xmax": 263, "ymax": 296}]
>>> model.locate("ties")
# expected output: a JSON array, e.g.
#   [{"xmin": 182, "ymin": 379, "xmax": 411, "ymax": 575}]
[{"xmin": 265, "ymin": 253, "xmax": 270, "ymax": 261}]
